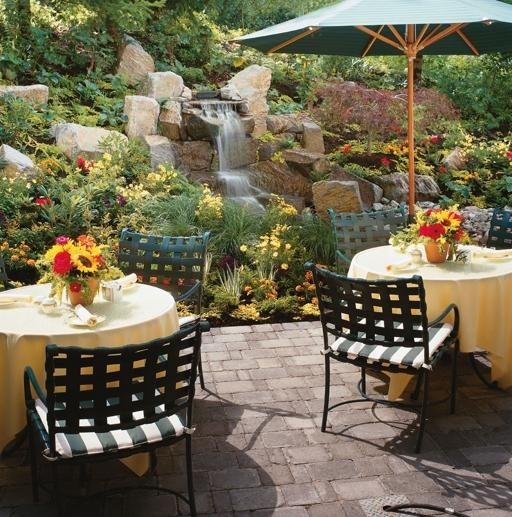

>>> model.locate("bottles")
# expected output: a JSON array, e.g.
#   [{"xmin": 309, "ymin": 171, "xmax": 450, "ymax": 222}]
[
  {"xmin": 411, "ymin": 251, "xmax": 422, "ymax": 264},
  {"xmin": 42, "ymin": 298, "xmax": 57, "ymax": 314}
]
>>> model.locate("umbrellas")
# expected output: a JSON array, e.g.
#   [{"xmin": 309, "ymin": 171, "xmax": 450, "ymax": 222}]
[{"xmin": 229, "ymin": 0, "xmax": 512, "ymax": 245}]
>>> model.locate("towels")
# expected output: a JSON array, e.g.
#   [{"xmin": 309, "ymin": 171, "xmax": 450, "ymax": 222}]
[{"xmin": 1, "ymin": 291, "xmax": 34, "ymax": 308}]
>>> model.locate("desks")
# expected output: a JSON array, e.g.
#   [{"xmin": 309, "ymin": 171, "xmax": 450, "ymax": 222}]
[
  {"xmin": 0, "ymin": 280, "xmax": 178, "ymax": 474},
  {"xmin": 341, "ymin": 239, "xmax": 507, "ymax": 411}
]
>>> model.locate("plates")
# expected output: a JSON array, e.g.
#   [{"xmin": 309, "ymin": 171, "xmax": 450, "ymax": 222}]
[{"xmin": 64, "ymin": 314, "xmax": 106, "ymax": 327}]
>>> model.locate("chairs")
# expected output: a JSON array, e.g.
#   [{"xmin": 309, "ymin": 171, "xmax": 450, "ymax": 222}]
[
  {"xmin": 325, "ymin": 204, "xmax": 408, "ymax": 340},
  {"xmin": 310, "ymin": 263, "xmax": 462, "ymax": 454},
  {"xmin": 113, "ymin": 227, "xmax": 211, "ymax": 390},
  {"xmin": 21, "ymin": 318, "xmax": 213, "ymax": 514}
]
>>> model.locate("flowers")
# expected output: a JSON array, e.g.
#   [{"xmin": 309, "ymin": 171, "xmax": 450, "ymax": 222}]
[{"xmin": 32, "ymin": 232, "xmax": 127, "ymax": 308}]
